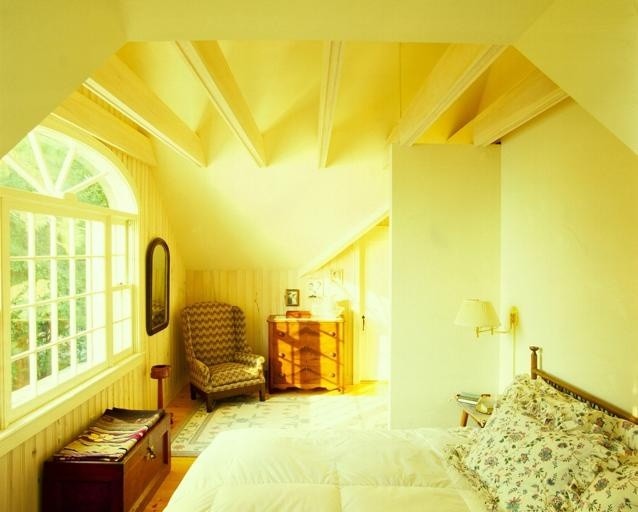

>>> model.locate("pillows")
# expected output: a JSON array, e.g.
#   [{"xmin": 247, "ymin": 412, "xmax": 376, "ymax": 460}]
[{"xmin": 450, "ymin": 374, "xmax": 638, "ymax": 510}]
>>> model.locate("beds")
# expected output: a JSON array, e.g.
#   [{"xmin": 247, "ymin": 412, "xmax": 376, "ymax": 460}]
[{"xmin": 160, "ymin": 345, "xmax": 638, "ymax": 511}]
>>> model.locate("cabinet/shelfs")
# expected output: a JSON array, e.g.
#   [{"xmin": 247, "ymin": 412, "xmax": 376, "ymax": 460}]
[{"xmin": 267, "ymin": 319, "xmax": 345, "ymax": 392}]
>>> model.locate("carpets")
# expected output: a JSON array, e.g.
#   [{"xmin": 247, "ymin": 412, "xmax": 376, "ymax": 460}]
[{"xmin": 169, "ymin": 395, "xmax": 387, "ymax": 456}]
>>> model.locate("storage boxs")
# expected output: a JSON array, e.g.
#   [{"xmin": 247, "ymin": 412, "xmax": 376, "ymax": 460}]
[{"xmin": 41, "ymin": 407, "xmax": 172, "ymax": 512}]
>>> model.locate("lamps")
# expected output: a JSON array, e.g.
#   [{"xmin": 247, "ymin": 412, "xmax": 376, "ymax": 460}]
[{"xmin": 453, "ymin": 298, "xmax": 517, "ymax": 338}]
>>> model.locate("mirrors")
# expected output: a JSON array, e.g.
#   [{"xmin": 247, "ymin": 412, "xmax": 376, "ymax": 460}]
[{"xmin": 146, "ymin": 237, "xmax": 171, "ymax": 335}]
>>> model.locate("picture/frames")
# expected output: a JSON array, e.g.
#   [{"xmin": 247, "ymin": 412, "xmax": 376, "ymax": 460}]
[{"xmin": 286, "ymin": 288, "xmax": 300, "ymax": 306}]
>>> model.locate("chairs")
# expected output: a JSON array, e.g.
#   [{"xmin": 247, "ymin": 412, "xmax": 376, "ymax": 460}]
[{"xmin": 180, "ymin": 300, "xmax": 266, "ymax": 413}]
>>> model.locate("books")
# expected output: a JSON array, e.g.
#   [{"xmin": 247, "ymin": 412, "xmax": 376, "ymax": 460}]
[{"xmin": 457, "ymin": 392, "xmax": 479, "ymax": 405}]
